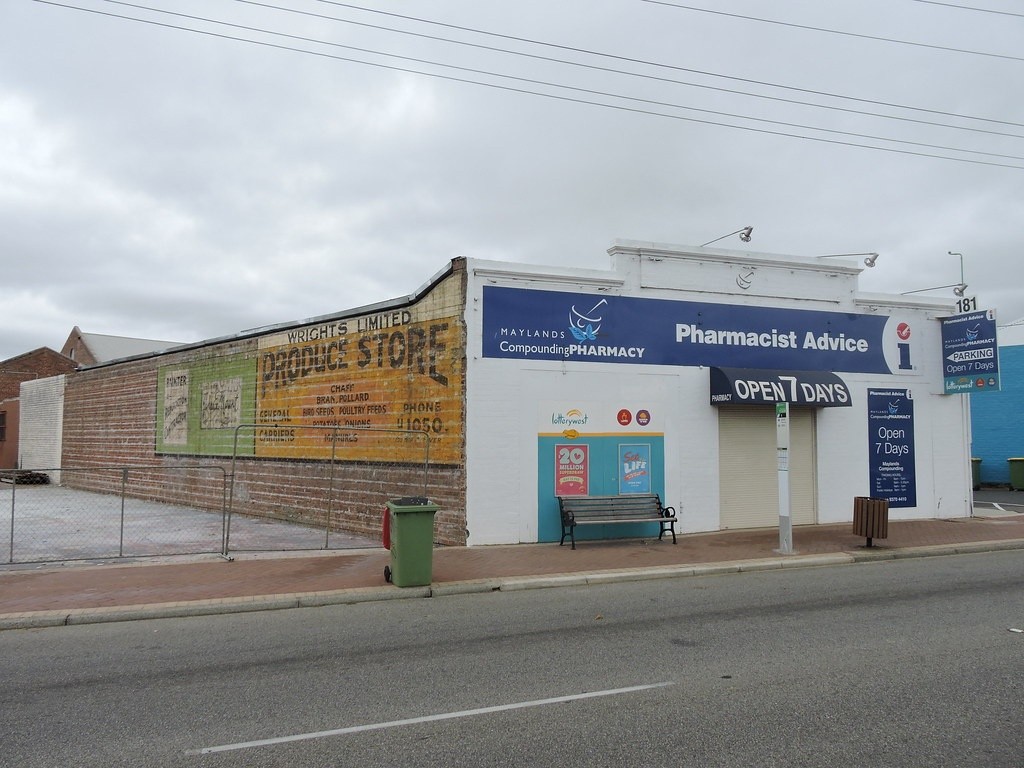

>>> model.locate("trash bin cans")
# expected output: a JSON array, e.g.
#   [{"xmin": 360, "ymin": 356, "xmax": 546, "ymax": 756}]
[
  {"xmin": 384, "ymin": 495, "xmax": 441, "ymax": 588},
  {"xmin": 971, "ymin": 457, "xmax": 983, "ymax": 491},
  {"xmin": 852, "ymin": 495, "xmax": 889, "ymax": 548},
  {"xmin": 1006, "ymin": 458, "xmax": 1024, "ymax": 491}
]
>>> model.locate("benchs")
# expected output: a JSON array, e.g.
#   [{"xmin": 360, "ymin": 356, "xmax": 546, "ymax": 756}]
[{"xmin": 558, "ymin": 493, "xmax": 678, "ymax": 551}]
